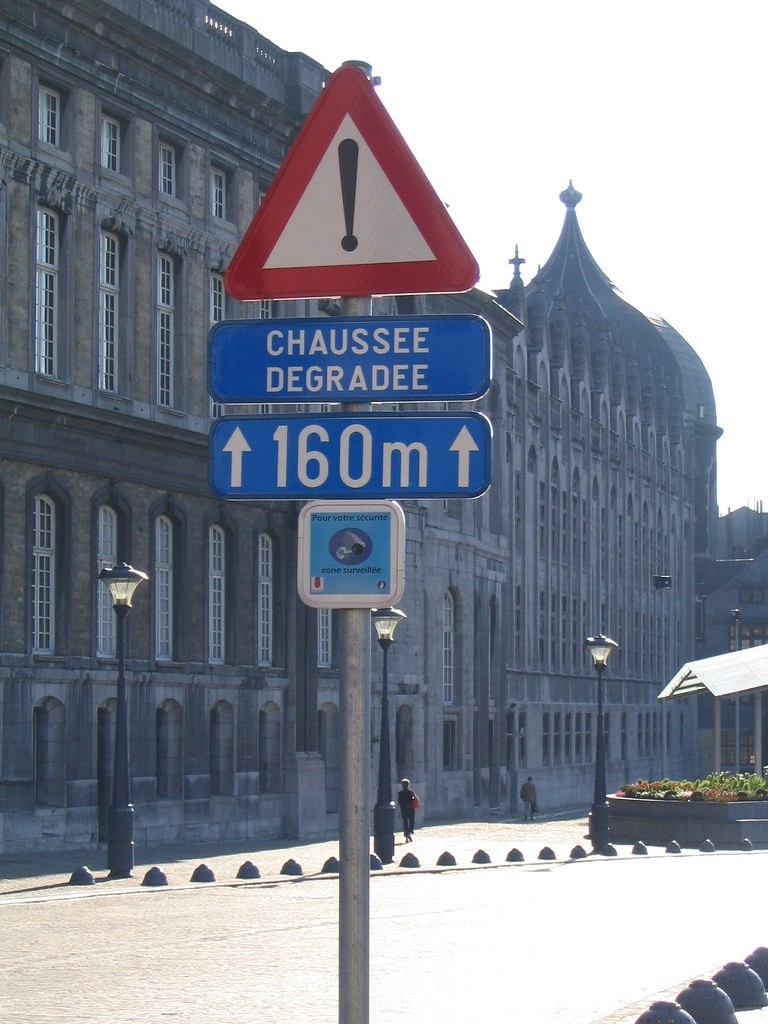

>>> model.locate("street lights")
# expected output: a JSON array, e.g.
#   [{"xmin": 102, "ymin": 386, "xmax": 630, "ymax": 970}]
[
  {"xmin": 98, "ymin": 561, "xmax": 149, "ymax": 879},
  {"xmin": 371, "ymin": 606, "xmax": 407, "ymax": 866},
  {"xmin": 730, "ymin": 608, "xmax": 742, "ymax": 772},
  {"xmin": 584, "ymin": 633, "xmax": 618, "ymax": 854}
]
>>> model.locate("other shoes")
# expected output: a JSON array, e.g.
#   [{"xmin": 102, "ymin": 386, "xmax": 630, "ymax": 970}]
[{"xmin": 406, "ymin": 835, "xmax": 413, "ymax": 843}]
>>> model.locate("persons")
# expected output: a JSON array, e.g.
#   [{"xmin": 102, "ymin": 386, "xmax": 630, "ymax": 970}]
[
  {"xmin": 520, "ymin": 776, "xmax": 537, "ymax": 822},
  {"xmin": 398, "ymin": 778, "xmax": 415, "ymax": 843}
]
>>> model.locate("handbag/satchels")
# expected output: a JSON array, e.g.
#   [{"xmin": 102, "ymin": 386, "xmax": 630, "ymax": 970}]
[
  {"xmin": 412, "ymin": 797, "xmax": 420, "ymax": 809},
  {"xmin": 533, "ymin": 802, "xmax": 538, "ymax": 812}
]
